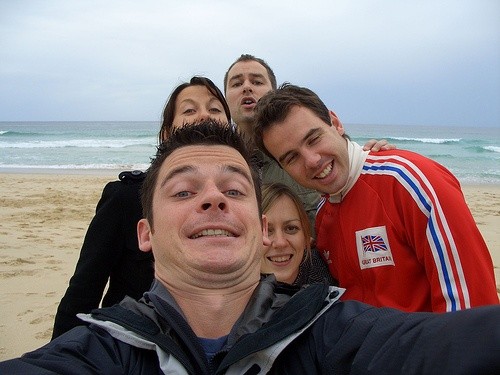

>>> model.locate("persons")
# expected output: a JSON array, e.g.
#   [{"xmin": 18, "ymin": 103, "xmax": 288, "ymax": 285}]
[
  {"xmin": 51, "ymin": 55, "xmax": 500, "ymax": 341},
  {"xmin": 0, "ymin": 118, "xmax": 500, "ymax": 375}
]
